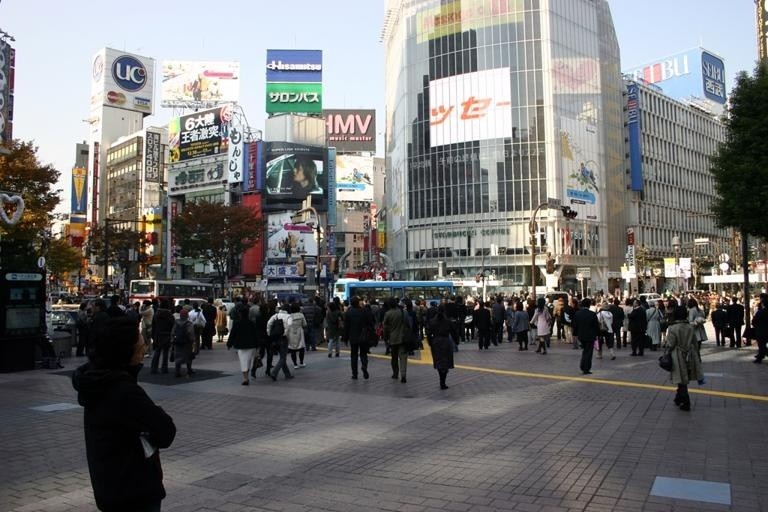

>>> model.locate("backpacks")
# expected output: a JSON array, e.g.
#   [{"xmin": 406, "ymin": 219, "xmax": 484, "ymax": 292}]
[{"xmin": 172, "ymin": 321, "xmax": 191, "ymax": 346}]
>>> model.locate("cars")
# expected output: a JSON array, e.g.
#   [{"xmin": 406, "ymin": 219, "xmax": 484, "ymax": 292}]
[
  {"xmin": 49, "ymin": 288, "xmax": 130, "ymax": 334},
  {"xmin": 637, "ymin": 292, "xmax": 662, "ymax": 307}
]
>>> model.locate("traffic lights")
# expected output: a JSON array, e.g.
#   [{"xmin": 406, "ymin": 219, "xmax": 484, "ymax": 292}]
[
  {"xmin": 478, "ymin": 267, "xmax": 493, "ymax": 305},
  {"xmin": 562, "ymin": 207, "xmax": 577, "ymax": 219}
]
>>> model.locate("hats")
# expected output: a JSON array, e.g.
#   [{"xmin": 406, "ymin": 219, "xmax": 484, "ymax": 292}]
[
  {"xmin": 180, "ymin": 309, "xmax": 189, "ymax": 317},
  {"xmin": 85, "ymin": 316, "xmax": 138, "ymax": 371}
]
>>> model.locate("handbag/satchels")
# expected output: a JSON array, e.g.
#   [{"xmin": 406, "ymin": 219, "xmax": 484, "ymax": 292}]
[
  {"xmin": 338, "ymin": 320, "xmax": 345, "ymax": 329},
  {"xmin": 743, "ymin": 323, "xmax": 755, "ymax": 340},
  {"xmin": 268, "ymin": 318, "xmax": 284, "ymax": 338},
  {"xmin": 361, "ymin": 325, "xmax": 379, "ymax": 347},
  {"xmin": 724, "ymin": 327, "xmax": 730, "ymax": 337},
  {"xmin": 659, "ymin": 317, "xmax": 666, "ymax": 332},
  {"xmin": 193, "ymin": 318, "xmax": 205, "ymax": 333},
  {"xmin": 658, "ymin": 353, "xmax": 673, "ymax": 372},
  {"xmin": 694, "ymin": 317, "xmax": 706, "ymax": 325},
  {"xmin": 402, "ymin": 330, "xmax": 420, "ymax": 353},
  {"xmin": 599, "ymin": 318, "xmax": 608, "ymax": 335},
  {"xmin": 545, "ymin": 311, "xmax": 552, "ymax": 326}
]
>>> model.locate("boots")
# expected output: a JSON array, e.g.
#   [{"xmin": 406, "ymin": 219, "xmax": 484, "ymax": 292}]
[
  {"xmin": 535, "ymin": 341, "xmax": 546, "ymax": 355},
  {"xmin": 596, "ymin": 351, "xmax": 602, "ymax": 359},
  {"xmin": 609, "ymin": 348, "xmax": 616, "ymax": 360}
]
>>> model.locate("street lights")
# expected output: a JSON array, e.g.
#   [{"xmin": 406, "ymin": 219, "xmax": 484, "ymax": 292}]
[
  {"xmin": 671, "ymin": 229, "xmax": 681, "ymax": 296},
  {"xmin": 755, "ymin": 258, "xmax": 767, "ymax": 295}
]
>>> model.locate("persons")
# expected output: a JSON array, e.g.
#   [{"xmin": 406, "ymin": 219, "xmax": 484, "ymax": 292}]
[
  {"xmin": 58, "ymin": 289, "xmax": 767, "ymax": 389},
  {"xmin": 282, "ymin": 231, "xmax": 295, "ymax": 264},
  {"xmin": 579, "ymin": 162, "xmax": 598, "ymax": 191},
  {"xmin": 289, "ymin": 158, "xmax": 323, "ymax": 194},
  {"xmin": 352, "ymin": 169, "xmax": 374, "ymax": 186},
  {"xmin": 662, "ymin": 305, "xmax": 705, "ymax": 411},
  {"xmin": 71, "ymin": 311, "xmax": 176, "ymax": 511}
]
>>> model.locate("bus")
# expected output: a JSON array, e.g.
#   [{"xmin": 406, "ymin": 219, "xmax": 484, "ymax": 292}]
[
  {"xmin": 328, "ymin": 278, "xmax": 454, "ymax": 310},
  {"xmin": 257, "ymin": 276, "xmax": 333, "ymax": 305},
  {"xmin": 130, "ymin": 279, "xmax": 215, "ymax": 311}
]
